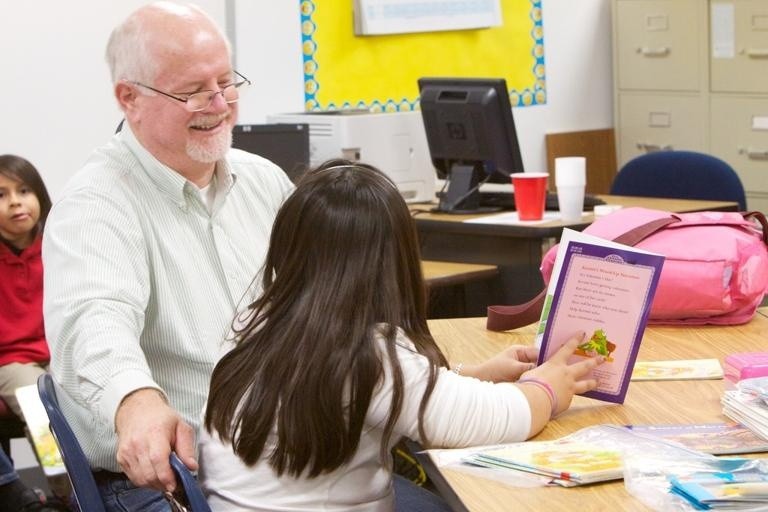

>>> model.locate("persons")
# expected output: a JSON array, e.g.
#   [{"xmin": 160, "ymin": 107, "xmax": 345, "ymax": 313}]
[
  {"xmin": 193, "ymin": 154, "xmax": 610, "ymax": 512},
  {"xmin": 40, "ymin": 0, "xmax": 427, "ymax": 512},
  {"xmin": 0, "ymin": 154, "xmax": 56, "ymax": 459}
]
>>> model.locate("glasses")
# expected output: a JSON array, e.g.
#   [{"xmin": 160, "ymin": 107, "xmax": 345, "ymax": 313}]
[{"xmin": 130, "ymin": 72, "xmax": 251, "ymax": 112}]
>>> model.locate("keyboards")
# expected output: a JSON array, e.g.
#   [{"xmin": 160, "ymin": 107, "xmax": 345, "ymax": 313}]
[{"xmin": 478, "ymin": 192, "xmax": 606, "ymax": 211}]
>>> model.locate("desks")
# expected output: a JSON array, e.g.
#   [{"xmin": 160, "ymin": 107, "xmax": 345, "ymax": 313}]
[{"xmin": 404, "ymin": 193, "xmax": 768, "ymax": 511}]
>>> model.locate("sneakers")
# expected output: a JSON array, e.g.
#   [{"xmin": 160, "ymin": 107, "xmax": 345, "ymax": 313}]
[{"xmin": 3, "ymin": 480, "xmax": 42, "ymax": 511}]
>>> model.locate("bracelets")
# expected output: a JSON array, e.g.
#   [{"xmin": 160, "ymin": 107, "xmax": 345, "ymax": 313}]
[
  {"xmin": 454, "ymin": 362, "xmax": 462, "ymax": 376},
  {"xmin": 516, "ymin": 377, "xmax": 558, "ymax": 420}
]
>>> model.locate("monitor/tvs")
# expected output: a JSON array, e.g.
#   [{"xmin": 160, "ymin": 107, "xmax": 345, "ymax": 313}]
[
  {"xmin": 418, "ymin": 77, "xmax": 525, "ymax": 215},
  {"xmin": 232, "ymin": 123, "xmax": 309, "ymax": 189}
]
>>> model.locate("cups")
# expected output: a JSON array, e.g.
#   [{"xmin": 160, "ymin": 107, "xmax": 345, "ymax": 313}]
[
  {"xmin": 509, "ymin": 173, "xmax": 550, "ymax": 222},
  {"xmin": 554, "ymin": 157, "xmax": 587, "ymax": 224}
]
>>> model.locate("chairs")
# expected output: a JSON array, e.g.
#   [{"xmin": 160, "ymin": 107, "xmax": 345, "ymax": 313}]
[
  {"xmin": 609, "ymin": 151, "xmax": 747, "ymax": 212},
  {"xmin": 34, "ymin": 373, "xmax": 212, "ymax": 512}
]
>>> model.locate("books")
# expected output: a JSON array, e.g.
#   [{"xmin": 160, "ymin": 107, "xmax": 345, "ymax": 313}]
[
  {"xmin": 536, "ymin": 228, "xmax": 665, "ymax": 405},
  {"xmin": 629, "ymin": 357, "xmax": 724, "ymax": 382},
  {"xmin": 352, "ymin": 0, "xmax": 502, "ymax": 38},
  {"xmin": 458, "ymin": 375, "xmax": 766, "ymax": 511}
]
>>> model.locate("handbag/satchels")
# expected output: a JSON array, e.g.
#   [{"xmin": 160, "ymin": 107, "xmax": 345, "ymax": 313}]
[{"xmin": 486, "ymin": 209, "xmax": 768, "ymax": 333}]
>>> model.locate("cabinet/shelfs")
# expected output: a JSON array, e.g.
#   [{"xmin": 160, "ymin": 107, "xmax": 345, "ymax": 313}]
[{"xmin": 606, "ymin": 0, "xmax": 767, "ymax": 216}]
